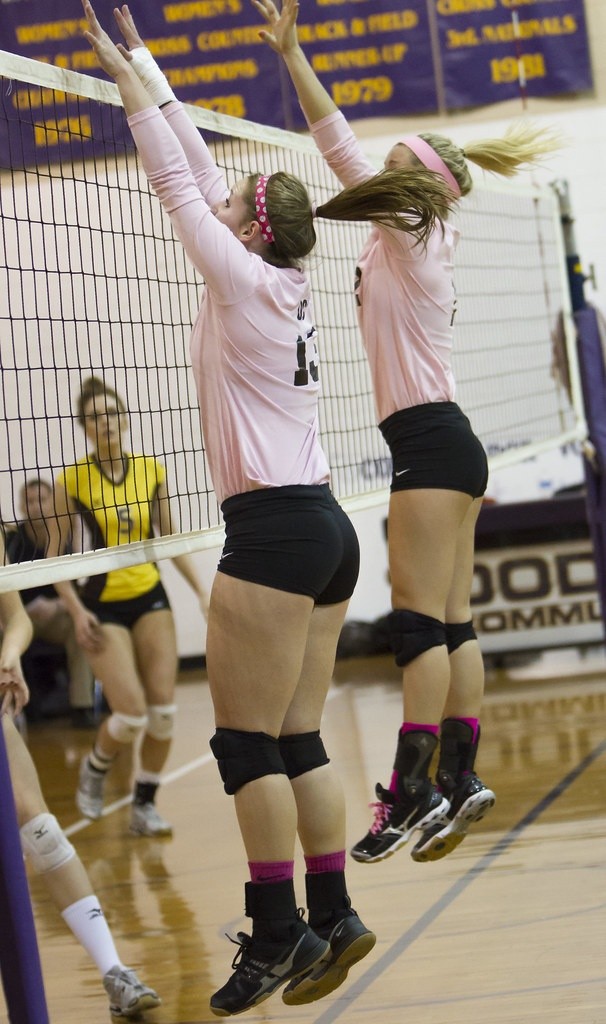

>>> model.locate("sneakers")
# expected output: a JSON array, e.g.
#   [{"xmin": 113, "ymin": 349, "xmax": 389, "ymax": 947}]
[
  {"xmin": 209, "ymin": 908, "xmax": 330, "ymax": 1017},
  {"xmin": 411, "ymin": 771, "xmax": 495, "ymax": 862},
  {"xmin": 75, "ymin": 752, "xmax": 103, "ymax": 820},
  {"xmin": 350, "ymin": 776, "xmax": 451, "ymax": 863},
  {"xmin": 102, "ymin": 965, "xmax": 161, "ymax": 1017},
  {"xmin": 282, "ymin": 906, "xmax": 376, "ymax": 1006},
  {"xmin": 129, "ymin": 805, "xmax": 172, "ymax": 838}
]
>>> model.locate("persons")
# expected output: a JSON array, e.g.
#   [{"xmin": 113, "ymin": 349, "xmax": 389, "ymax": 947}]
[
  {"xmin": 1, "ymin": 480, "xmax": 102, "ymax": 730},
  {"xmin": 47, "ymin": 377, "xmax": 212, "ymax": 838},
  {"xmin": 0, "ymin": 533, "xmax": 162, "ymax": 1015},
  {"xmin": 253, "ymin": 0, "xmax": 497, "ymax": 864},
  {"xmin": 79, "ymin": 0, "xmax": 377, "ymax": 1017}
]
